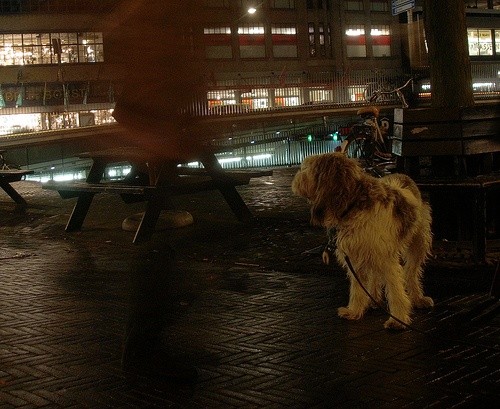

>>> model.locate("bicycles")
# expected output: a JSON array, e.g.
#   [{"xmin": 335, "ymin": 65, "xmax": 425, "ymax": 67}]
[{"xmin": 335, "ymin": 78, "xmax": 414, "ymax": 169}]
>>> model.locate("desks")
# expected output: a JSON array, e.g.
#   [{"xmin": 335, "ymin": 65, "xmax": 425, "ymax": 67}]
[{"xmin": 64, "ymin": 147, "xmax": 254, "ymax": 244}]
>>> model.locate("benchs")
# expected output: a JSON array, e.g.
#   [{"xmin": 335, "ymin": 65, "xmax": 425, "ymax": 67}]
[
  {"xmin": 43, "ymin": 180, "xmax": 160, "ymax": 204},
  {"xmin": 176, "ymin": 167, "xmax": 273, "ymax": 186},
  {"xmin": 0, "ymin": 170, "xmax": 34, "ymax": 204},
  {"xmin": 362, "ymin": 103, "xmax": 500, "ymax": 261}
]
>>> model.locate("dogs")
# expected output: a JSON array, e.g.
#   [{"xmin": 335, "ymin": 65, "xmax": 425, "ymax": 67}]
[{"xmin": 292, "ymin": 152, "xmax": 435, "ymax": 332}]
